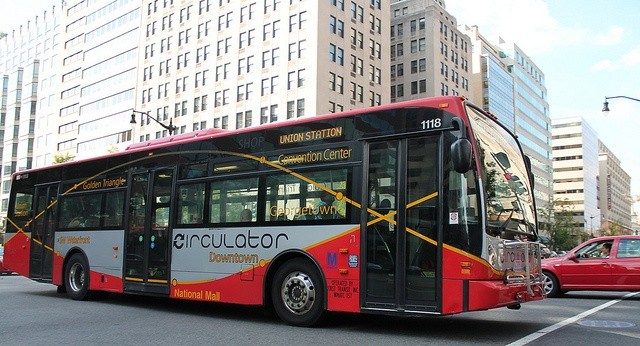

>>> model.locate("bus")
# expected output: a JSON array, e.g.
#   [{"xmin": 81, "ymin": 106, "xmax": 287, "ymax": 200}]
[{"xmin": 3, "ymin": 96, "xmax": 544, "ymax": 325}]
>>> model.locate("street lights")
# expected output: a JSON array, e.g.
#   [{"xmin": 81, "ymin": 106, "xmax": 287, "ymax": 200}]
[
  {"xmin": 131, "ymin": 110, "xmax": 176, "ymax": 135},
  {"xmin": 590, "ymin": 214, "xmax": 605, "ymax": 235},
  {"xmin": 602, "ymin": 96, "xmax": 640, "ymax": 111}
]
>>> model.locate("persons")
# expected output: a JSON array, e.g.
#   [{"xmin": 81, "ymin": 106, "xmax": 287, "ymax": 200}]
[
  {"xmin": 240, "ymin": 209, "xmax": 252, "ymax": 222},
  {"xmin": 192, "ymin": 213, "xmax": 202, "ymax": 224},
  {"xmin": 583, "ymin": 243, "xmax": 612, "ymax": 258},
  {"xmin": 371, "ymin": 199, "xmax": 395, "ymax": 246},
  {"xmin": 316, "ymin": 190, "xmax": 344, "ymax": 220}
]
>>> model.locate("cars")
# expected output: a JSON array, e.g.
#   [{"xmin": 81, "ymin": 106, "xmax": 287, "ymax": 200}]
[{"xmin": 542, "ymin": 235, "xmax": 640, "ymax": 296}]
